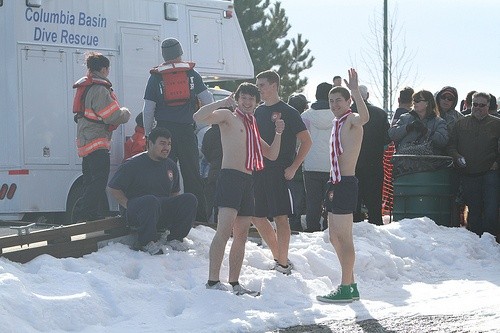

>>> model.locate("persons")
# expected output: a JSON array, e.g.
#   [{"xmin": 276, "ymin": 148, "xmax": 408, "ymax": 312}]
[
  {"xmin": 350, "ymin": 83, "xmax": 392, "ymax": 226},
  {"xmin": 143, "ymin": 39, "xmax": 214, "ymax": 225},
  {"xmin": 193, "ymin": 83, "xmax": 285, "ymax": 297},
  {"xmin": 195, "ymin": 106, "xmax": 234, "ymax": 222},
  {"xmin": 72, "ymin": 51, "xmax": 131, "ymax": 221},
  {"xmin": 247, "ymin": 71, "xmax": 312, "ymax": 276},
  {"xmin": 111, "ymin": 127, "xmax": 197, "ymax": 255},
  {"xmin": 316, "ymin": 68, "xmax": 369, "ymax": 302},
  {"xmin": 124, "ymin": 112, "xmax": 147, "ymax": 161},
  {"xmin": 287, "ymin": 76, "xmax": 342, "ymax": 234},
  {"xmin": 387, "ymin": 85, "xmax": 500, "ymax": 244}
]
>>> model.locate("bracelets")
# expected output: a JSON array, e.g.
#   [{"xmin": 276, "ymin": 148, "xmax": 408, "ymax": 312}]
[{"xmin": 276, "ymin": 131, "xmax": 282, "ymax": 136}]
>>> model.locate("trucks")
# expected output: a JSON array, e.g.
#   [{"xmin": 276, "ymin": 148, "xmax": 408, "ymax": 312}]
[{"xmin": 0, "ymin": 0, "xmax": 256, "ymax": 227}]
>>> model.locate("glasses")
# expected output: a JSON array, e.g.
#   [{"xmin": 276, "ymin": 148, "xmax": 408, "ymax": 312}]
[
  {"xmin": 413, "ymin": 99, "xmax": 428, "ymax": 103},
  {"xmin": 472, "ymin": 101, "xmax": 489, "ymax": 107},
  {"xmin": 441, "ymin": 95, "xmax": 453, "ymax": 100}
]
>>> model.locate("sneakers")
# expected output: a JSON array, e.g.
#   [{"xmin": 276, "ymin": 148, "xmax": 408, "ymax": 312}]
[
  {"xmin": 275, "ymin": 257, "xmax": 293, "ymax": 270},
  {"xmin": 316, "ymin": 284, "xmax": 353, "ymax": 303},
  {"xmin": 350, "ymin": 283, "xmax": 360, "ymax": 300},
  {"xmin": 270, "ymin": 263, "xmax": 292, "ymax": 276},
  {"xmin": 204, "ymin": 281, "xmax": 228, "ymax": 291},
  {"xmin": 165, "ymin": 237, "xmax": 194, "ymax": 251},
  {"xmin": 232, "ymin": 285, "xmax": 260, "ymax": 297},
  {"xmin": 142, "ymin": 241, "xmax": 163, "ymax": 255}
]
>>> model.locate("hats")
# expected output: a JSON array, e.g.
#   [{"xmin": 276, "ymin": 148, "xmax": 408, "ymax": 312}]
[
  {"xmin": 161, "ymin": 38, "xmax": 183, "ymax": 61},
  {"xmin": 287, "ymin": 93, "xmax": 311, "ymax": 108}
]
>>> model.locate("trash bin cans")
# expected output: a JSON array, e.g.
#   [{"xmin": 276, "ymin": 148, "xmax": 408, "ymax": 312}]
[{"xmin": 391, "ymin": 153, "xmax": 455, "ymax": 227}]
